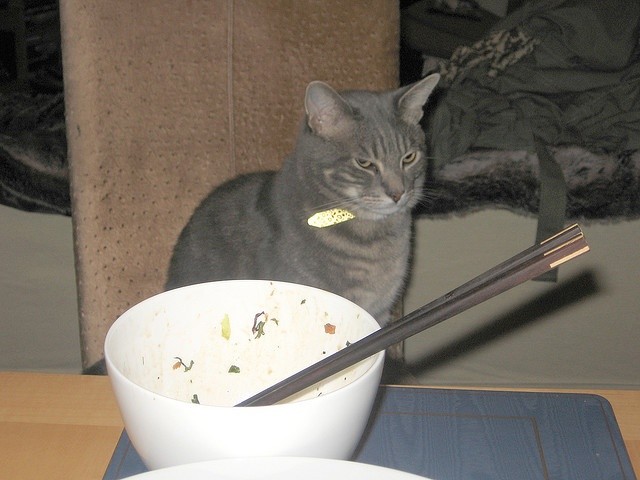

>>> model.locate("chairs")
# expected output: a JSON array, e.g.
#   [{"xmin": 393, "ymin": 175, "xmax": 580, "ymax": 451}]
[{"xmin": 58, "ymin": 3, "xmax": 401, "ymax": 373}]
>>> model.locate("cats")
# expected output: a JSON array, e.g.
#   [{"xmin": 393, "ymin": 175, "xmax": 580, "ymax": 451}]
[{"xmin": 81, "ymin": 72, "xmax": 441, "ymax": 384}]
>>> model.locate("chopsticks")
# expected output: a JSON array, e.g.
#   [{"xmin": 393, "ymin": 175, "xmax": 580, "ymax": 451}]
[{"xmin": 236, "ymin": 222, "xmax": 592, "ymax": 409}]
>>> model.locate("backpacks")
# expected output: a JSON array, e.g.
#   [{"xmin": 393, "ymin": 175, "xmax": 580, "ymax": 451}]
[{"xmin": 411, "ymin": 0, "xmax": 639, "ymax": 283}]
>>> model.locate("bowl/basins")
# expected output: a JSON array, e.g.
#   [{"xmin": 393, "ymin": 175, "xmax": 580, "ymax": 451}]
[{"xmin": 104, "ymin": 278, "xmax": 385, "ymax": 472}]
